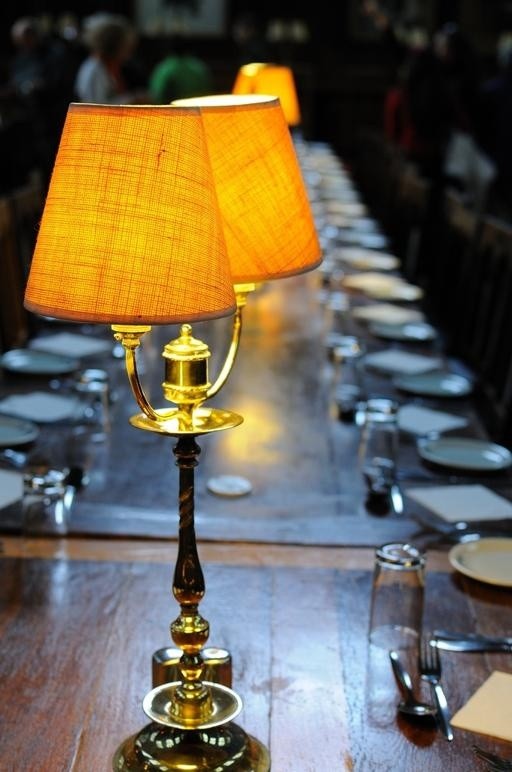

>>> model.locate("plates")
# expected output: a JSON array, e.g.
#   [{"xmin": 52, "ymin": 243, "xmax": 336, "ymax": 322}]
[
  {"xmin": 448, "ymin": 536, "xmax": 512, "ymax": 588},
  {"xmin": 0, "ymin": 414, "xmax": 40, "ymax": 447},
  {"xmin": 292, "ymin": 125, "xmax": 473, "ymax": 396},
  {"xmin": 417, "ymin": 436, "xmax": 511, "ymax": 471},
  {"xmin": 0, "ymin": 347, "xmax": 80, "ymax": 375}
]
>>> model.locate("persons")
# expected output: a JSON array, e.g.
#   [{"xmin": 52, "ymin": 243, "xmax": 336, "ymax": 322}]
[
  {"xmin": 374, "ymin": 36, "xmax": 512, "ymax": 289},
  {"xmin": 2, "ymin": 1, "xmax": 208, "ymax": 186}
]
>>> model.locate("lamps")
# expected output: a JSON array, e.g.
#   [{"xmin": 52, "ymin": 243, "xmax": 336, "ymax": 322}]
[{"xmin": 23, "ymin": 88, "xmax": 326, "ymax": 772}]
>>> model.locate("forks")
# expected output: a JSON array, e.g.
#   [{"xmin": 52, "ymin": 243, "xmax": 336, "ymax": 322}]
[{"xmin": 416, "ymin": 642, "xmax": 454, "ymax": 742}]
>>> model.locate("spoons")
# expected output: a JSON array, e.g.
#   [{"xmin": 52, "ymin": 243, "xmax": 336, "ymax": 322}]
[{"xmin": 388, "ymin": 648, "xmax": 438, "ymax": 727}]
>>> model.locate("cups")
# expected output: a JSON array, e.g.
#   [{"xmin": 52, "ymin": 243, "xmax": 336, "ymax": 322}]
[
  {"xmin": 366, "ymin": 542, "xmax": 428, "ymax": 650},
  {"xmin": 360, "ymin": 397, "xmax": 401, "ymax": 475},
  {"xmin": 71, "ymin": 368, "xmax": 111, "ymax": 444}
]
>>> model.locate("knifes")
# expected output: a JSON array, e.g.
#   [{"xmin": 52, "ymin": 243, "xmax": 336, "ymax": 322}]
[{"xmin": 429, "ymin": 636, "xmax": 512, "ymax": 653}]
